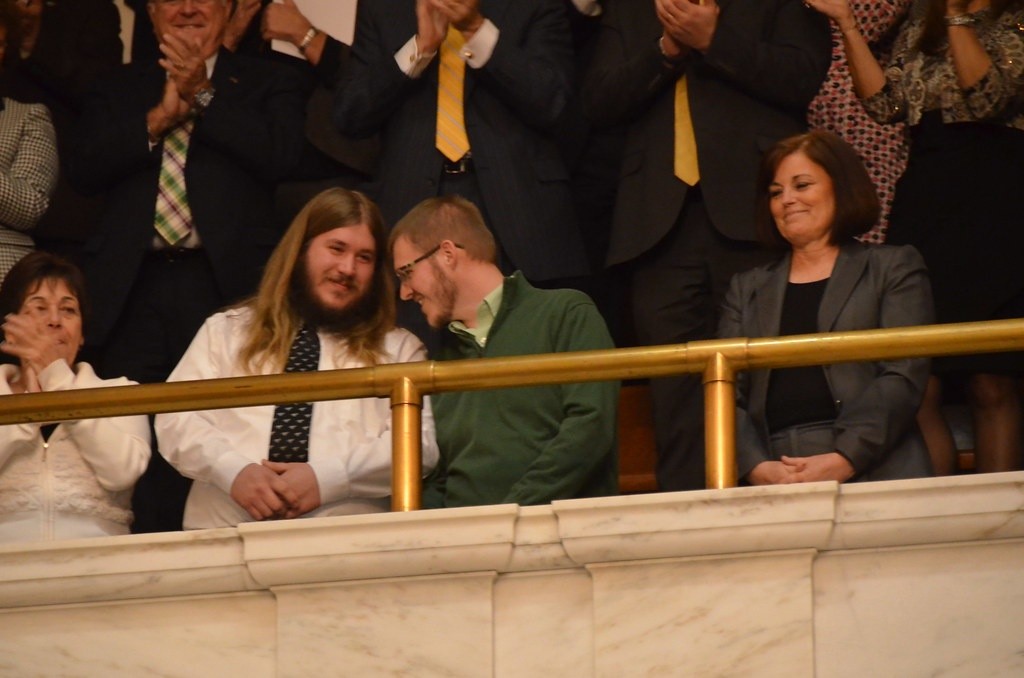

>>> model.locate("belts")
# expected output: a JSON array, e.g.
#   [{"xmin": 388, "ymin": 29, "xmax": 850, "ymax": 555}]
[{"xmin": 144, "ymin": 246, "xmax": 211, "ymax": 269}]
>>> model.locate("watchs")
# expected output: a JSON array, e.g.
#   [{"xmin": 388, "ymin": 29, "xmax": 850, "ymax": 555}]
[{"xmin": 191, "ymin": 83, "xmax": 216, "ymax": 110}]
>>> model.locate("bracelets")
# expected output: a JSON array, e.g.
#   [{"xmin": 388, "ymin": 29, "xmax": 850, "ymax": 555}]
[
  {"xmin": 944, "ymin": 13, "xmax": 982, "ymax": 27},
  {"xmin": 298, "ymin": 27, "xmax": 319, "ymax": 53}
]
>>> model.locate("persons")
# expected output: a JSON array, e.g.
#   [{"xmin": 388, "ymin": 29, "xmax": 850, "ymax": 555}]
[
  {"xmin": 802, "ymin": 0, "xmax": 913, "ymax": 243},
  {"xmin": 150, "ymin": 190, "xmax": 441, "ymax": 531},
  {"xmin": 334, "ymin": 1, "xmax": 595, "ymax": 352},
  {"xmin": 0, "ymin": 251, "xmax": 151, "ymax": 554},
  {"xmin": 6, "ymin": 0, "xmax": 123, "ymax": 158},
  {"xmin": 807, "ymin": 1, "xmax": 1022, "ymax": 474},
  {"xmin": 388, "ymin": 193, "xmax": 621, "ymax": 511},
  {"xmin": 593, "ymin": 0, "xmax": 834, "ymax": 494},
  {"xmin": 716, "ymin": 126, "xmax": 941, "ymax": 486},
  {"xmin": 86, "ymin": 1, "xmax": 262, "ymax": 390},
  {"xmin": 217, "ymin": 1, "xmax": 359, "ymax": 259},
  {"xmin": 0, "ymin": 3, "xmax": 56, "ymax": 292}
]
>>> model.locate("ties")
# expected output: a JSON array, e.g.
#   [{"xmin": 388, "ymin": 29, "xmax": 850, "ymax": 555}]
[
  {"xmin": 674, "ymin": 71, "xmax": 701, "ymax": 187},
  {"xmin": 154, "ymin": 107, "xmax": 198, "ymax": 243},
  {"xmin": 436, "ymin": 20, "xmax": 470, "ymax": 163}
]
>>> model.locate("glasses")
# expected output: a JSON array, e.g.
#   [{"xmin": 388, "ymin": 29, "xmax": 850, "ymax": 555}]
[{"xmin": 394, "ymin": 243, "xmax": 465, "ymax": 283}]
